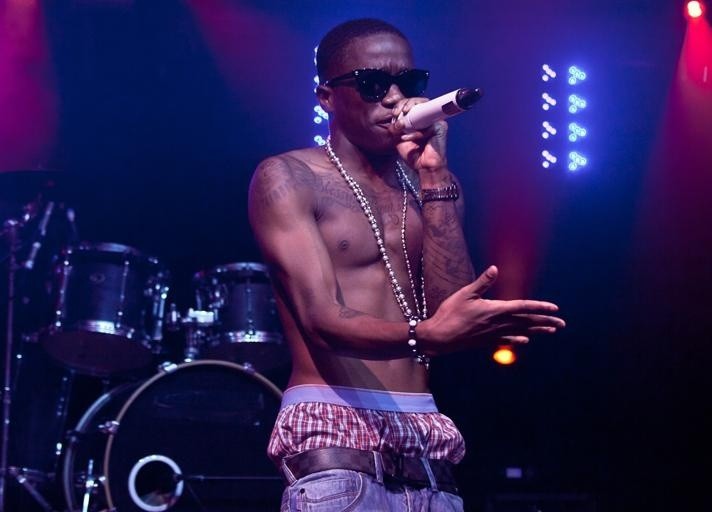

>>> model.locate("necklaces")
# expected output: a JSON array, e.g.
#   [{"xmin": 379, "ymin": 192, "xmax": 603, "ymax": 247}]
[{"xmin": 325, "ymin": 129, "xmax": 428, "ymax": 321}]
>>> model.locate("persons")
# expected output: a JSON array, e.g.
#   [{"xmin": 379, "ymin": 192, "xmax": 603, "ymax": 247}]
[{"xmin": 246, "ymin": 18, "xmax": 568, "ymax": 512}]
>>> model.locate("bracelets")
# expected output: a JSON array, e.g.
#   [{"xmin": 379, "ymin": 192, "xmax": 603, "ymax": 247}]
[
  {"xmin": 407, "ymin": 316, "xmax": 432, "ymax": 372},
  {"xmin": 420, "ymin": 182, "xmax": 459, "ymax": 202}
]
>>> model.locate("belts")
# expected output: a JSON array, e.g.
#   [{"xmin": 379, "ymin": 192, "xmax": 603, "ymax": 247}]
[{"xmin": 278, "ymin": 446, "xmax": 468, "ymax": 500}]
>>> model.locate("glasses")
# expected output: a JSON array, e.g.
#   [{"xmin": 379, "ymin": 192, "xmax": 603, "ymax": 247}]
[{"xmin": 323, "ymin": 68, "xmax": 431, "ymax": 103}]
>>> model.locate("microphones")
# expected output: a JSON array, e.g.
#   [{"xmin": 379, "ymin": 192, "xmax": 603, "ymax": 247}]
[{"xmin": 391, "ymin": 87, "xmax": 485, "ymax": 134}]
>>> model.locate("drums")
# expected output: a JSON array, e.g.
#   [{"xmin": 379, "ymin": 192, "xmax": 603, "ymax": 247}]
[
  {"xmin": 2, "ymin": 200, "xmax": 80, "ymax": 285},
  {"xmin": 63, "ymin": 361, "xmax": 286, "ymax": 510},
  {"xmin": 194, "ymin": 263, "xmax": 283, "ymax": 355},
  {"xmin": 38, "ymin": 239, "xmax": 171, "ymax": 373}
]
